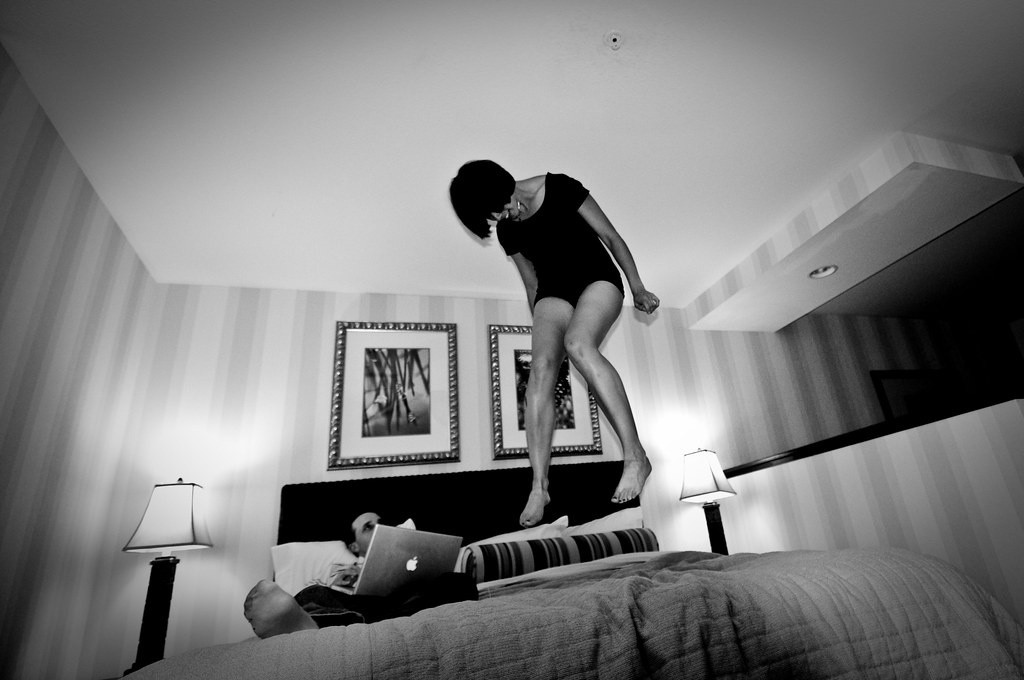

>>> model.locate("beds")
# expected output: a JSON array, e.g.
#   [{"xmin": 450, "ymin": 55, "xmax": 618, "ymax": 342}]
[{"xmin": 121, "ymin": 461, "xmax": 1024, "ymax": 680}]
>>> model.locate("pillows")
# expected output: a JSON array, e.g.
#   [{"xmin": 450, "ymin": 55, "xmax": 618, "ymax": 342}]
[
  {"xmin": 269, "ymin": 517, "xmax": 416, "ymax": 598},
  {"xmin": 455, "ymin": 504, "xmax": 661, "ymax": 584}
]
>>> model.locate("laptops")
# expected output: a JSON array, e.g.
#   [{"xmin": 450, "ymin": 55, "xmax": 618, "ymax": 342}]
[{"xmin": 330, "ymin": 525, "xmax": 464, "ymax": 596}]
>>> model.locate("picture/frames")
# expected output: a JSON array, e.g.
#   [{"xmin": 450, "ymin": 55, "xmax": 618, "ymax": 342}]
[
  {"xmin": 489, "ymin": 325, "xmax": 602, "ymax": 460},
  {"xmin": 328, "ymin": 322, "xmax": 460, "ymax": 470}
]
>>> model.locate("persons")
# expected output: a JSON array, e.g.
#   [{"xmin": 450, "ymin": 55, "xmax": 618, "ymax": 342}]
[
  {"xmin": 241, "ymin": 512, "xmax": 479, "ymax": 639},
  {"xmin": 449, "ymin": 160, "xmax": 659, "ymax": 526}
]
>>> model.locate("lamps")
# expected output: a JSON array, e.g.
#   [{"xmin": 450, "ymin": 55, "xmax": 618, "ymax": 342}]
[
  {"xmin": 679, "ymin": 448, "xmax": 738, "ymax": 555},
  {"xmin": 123, "ymin": 477, "xmax": 213, "ymax": 676}
]
